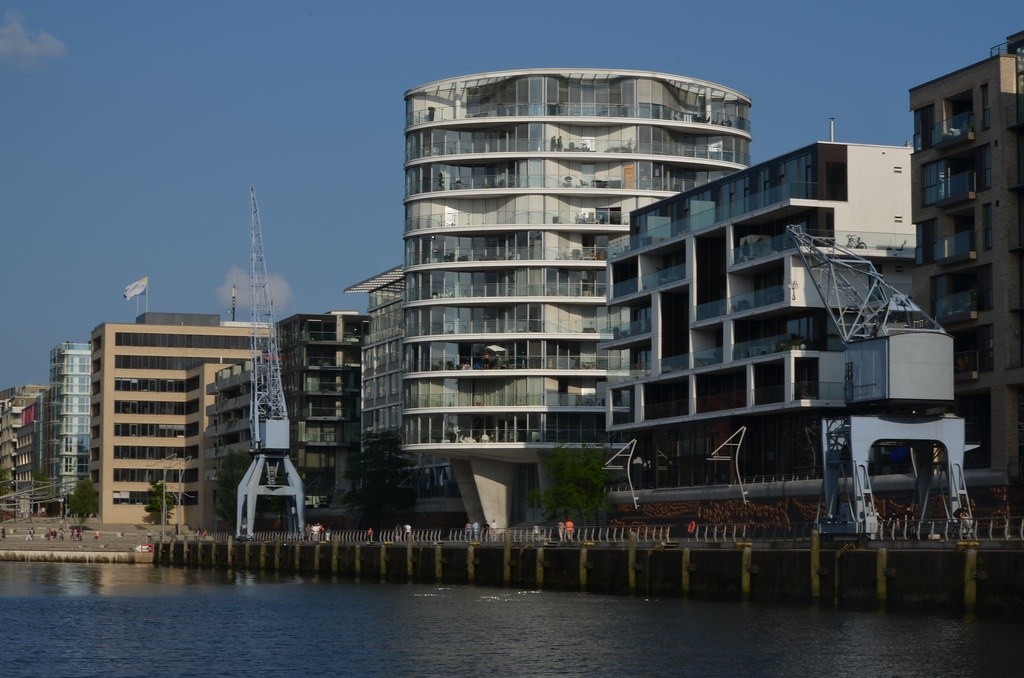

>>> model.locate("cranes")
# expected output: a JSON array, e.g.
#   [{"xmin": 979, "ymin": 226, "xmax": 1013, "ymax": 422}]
[
  {"xmin": 237, "ymin": 185, "xmax": 306, "ymax": 537},
  {"xmin": 781, "ymin": 224, "xmax": 974, "ymax": 540}
]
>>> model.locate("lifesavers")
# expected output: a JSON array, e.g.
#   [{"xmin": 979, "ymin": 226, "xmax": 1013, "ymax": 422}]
[{"xmin": 687, "ymin": 521, "xmax": 696, "ymax": 533}]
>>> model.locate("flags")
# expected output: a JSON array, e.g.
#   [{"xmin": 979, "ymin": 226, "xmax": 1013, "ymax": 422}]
[{"xmin": 124, "ymin": 277, "xmax": 147, "ymax": 300}]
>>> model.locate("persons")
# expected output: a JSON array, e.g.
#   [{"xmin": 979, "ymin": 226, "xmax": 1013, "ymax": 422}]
[
  {"xmin": 959, "ymin": 507, "xmax": 969, "ymax": 518},
  {"xmin": 481, "ymin": 520, "xmax": 489, "ymax": 541},
  {"xmin": 41, "ymin": 506, "xmax": 45, "ymax": 516},
  {"xmin": 558, "ymin": 520, "xmax": 565, "ymax": 542},
  {"xmin": 875, "ymin": 507, "xmax": 883, "ymax": 519},
  {"xmin": 551, "ymin": 136, "xmax": 563, "ymax": 151},
  {"xmin": 904, "ymin": 506, "xmax": 914, "ymax": 520},
  {"xmin": 147, "ymin": 530, "xmax": 152, "ymax": 542},
  {"xmin": 886, "ymin": 510, "xmax": 900, "ymax": 528},
  {"xmin": 93, "ymin": 529, "xmax": 100, "ymax": 539},
  {"xmin": 24, "ymin": 526, "xmax": 35, "ymax": 542},
  {"xmin": 45, "ymin": 528, "xmax": 64, "ymax": 540},
  {"xmin": 464, "ymin": 521, "xmax": 472, "ymax": 537},
  {"xmin": 70, "ymin": 527, "xmax": 80, "ymax": 539},
  {"xmin": 472, "ymin": 521, "xmax": 480, "ymax": 540},
  {"xmin": 483, "ymin": 349, "xmax": 490, "ymax": 368},
  {"xmin": 21, "ymin": 509, "xmax": 24, "ymax": 516},
  {"xmin": 305, "ymin": 522, "xmax": 331, "ymax": 540},
  {"xmin": 565, "ymin": 517, "xmax": 574, "ymax": 541}
]
